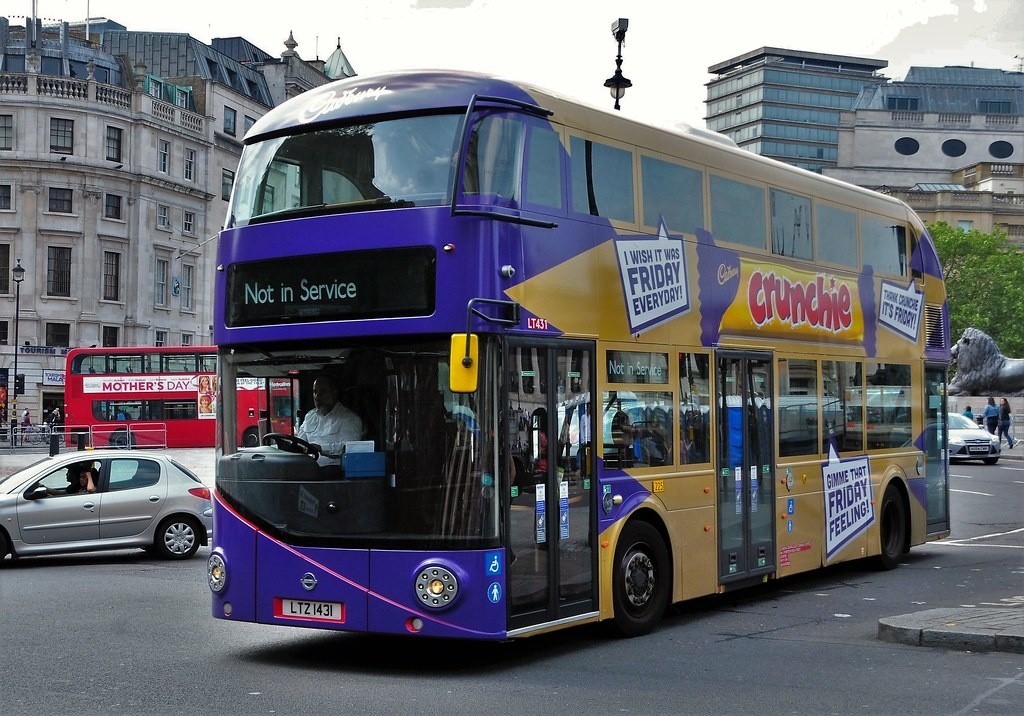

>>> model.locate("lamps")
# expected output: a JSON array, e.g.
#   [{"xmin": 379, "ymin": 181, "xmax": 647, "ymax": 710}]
[
  {"xmin": 61, "ymin": 157, "xmax": 67, "ymax": 161},
  {"xmin": 25, "ymin": 340, "xmax": 30, "ymax": 345},
  {"xmin": 89, "ymin": 345, "xmax": 97, "ymax": 348},
  {"xmin": 116, "ymin": 164, "xmax": 124, "ymax": 170}
]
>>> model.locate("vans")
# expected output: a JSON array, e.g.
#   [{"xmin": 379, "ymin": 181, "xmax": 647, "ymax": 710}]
[{"xmin": 846, "ymin": 389, "xmax": 910, "ymax": 442}]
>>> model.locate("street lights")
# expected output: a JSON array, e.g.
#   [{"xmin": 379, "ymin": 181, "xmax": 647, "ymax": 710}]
[
  {"xmin": 603, "ymin": 18, "xmax": 633, "ymax": 110},
  {"xmin": 11, "ymin": 258, "xmax": 26, "ymax": 448}
]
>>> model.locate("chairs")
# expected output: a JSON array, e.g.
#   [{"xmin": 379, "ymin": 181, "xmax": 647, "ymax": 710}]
[
  {"xmin": 88, "ymin": 366, "xmax": 208, "ymax": 373},
  {"xmin": 90, "ymin": 467, "xmax": 98, "ymax": 485}
]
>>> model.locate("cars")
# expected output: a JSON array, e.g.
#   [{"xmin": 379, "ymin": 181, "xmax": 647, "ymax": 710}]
[
  {"xmin": 0, "ymin": 450, "xmax": 213, "ymax": 565},
  {"xmin": 937, "ymin": 412, "xmax": 1002, "ymax": 465}
]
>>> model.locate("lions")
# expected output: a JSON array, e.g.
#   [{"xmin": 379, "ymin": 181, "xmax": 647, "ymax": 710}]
[{"xmin": 948, "ymin": 327, "xmax": 1024, "ymax": 398}]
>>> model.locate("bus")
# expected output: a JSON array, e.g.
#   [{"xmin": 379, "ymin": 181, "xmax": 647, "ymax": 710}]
[
  {"xmin": 208, "ymin": 69, "xmax": 952, "ymax": 640},
  {"xmin": 64, "ymin": 346, "xmax": 300, "ymax": 448}
]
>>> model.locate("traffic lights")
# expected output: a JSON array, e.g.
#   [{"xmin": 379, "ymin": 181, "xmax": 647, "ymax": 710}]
[{"xmin": 679, "ymin": 353, "xmax": 687, "ymax": 378}]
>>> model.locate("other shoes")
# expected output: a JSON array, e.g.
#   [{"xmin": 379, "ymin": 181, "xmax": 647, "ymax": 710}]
[{"xmin": 1009, "ymin": 444, "xmax": 1013, "ymax": 449}]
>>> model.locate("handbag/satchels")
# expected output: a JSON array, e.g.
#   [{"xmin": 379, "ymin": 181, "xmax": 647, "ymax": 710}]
[{"xmin": 1008, "ymin": 413, "xmax": 1013, "ymax": 420}]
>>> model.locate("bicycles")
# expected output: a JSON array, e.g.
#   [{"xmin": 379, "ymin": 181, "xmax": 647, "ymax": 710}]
[{"xmin": 29, "ymin": 422, "xmax": 64, "ymax": 445}]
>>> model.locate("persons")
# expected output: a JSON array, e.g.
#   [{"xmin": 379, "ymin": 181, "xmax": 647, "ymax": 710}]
[
  {"xmin": 21, "ymin": 408, "xmax": 31, "ymax": 443},
  {"xmin": 483, "ymin": 429, "xmax": 517, "ymax": 568},
  {"xmin": 0, "ymin": 409, "xmax": 8, "ymax": 441},
  {"xmin": 103, "ymin": 409, "xmax": 131, "ymax": 420},
  {"xmin": 998, "ymin": 398, "xmax": 1013, "ymax": 449},
  {"xmin": 529, "ymin": 406, "xmax": 566, "ymax": 550},
  {"xmin": 38, "ymin": 464, "xmax": 99, "ymax": 496},
  {"xmin": 618, "ymin": 409, "xmax": 703, "ymax": 466},
  {"xmin": 200, "ymin": 376, "xmax": 217, "ymax": 414},
  {"xmin": 962, "ymin": 406, "xmax": 973, "ymax": 420},
  {"xmin": 281, "ymin": 377, "xmax": 363, "ymax": 514},
  {"xmin": 44, "ymin": 409, "xmax": 60, "ymax": 444},
  {"xmin": 982, "ymin": 397, "xmax": 999, "ymax": 435}
]
